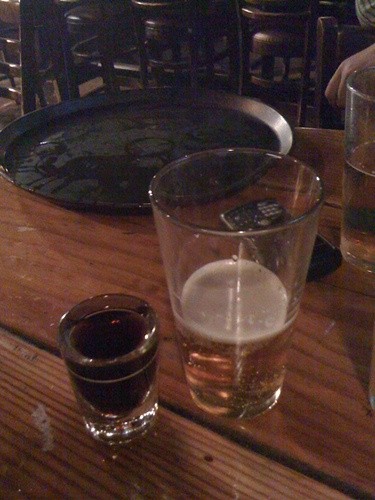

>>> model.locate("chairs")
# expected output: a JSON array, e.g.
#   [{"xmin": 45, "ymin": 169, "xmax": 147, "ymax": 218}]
[{"xmin": 0, "ymin": 0, "xmax": 375, "ymax": 131}]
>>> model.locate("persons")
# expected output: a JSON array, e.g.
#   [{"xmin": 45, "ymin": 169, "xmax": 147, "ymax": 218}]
[{"xmin": 325, "ymin": 0, "xmax": 375, "ymax": 110}]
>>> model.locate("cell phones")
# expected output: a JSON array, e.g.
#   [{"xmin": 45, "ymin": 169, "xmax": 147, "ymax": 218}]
[{"xmin": 220, "ymin": 197, "xmax": 343, "ymax": 281}]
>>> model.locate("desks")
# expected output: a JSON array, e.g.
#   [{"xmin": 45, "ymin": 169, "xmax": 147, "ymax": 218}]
[{"xmin": 0, "ymin": 127, "xmax": 374, "ymax": 500}]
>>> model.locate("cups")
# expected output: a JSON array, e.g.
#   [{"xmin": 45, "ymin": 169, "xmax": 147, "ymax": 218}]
[
  {"xmin": 148, "ymin": 146, "xmax": 324, "ymax": 421},
  {"xmin": 340, "ymin": 66, "xmax": 375, "ymax": 275},
  {"xmin": 57, "ymin": 293, "xmax": 163, "ymax": 446}
]
